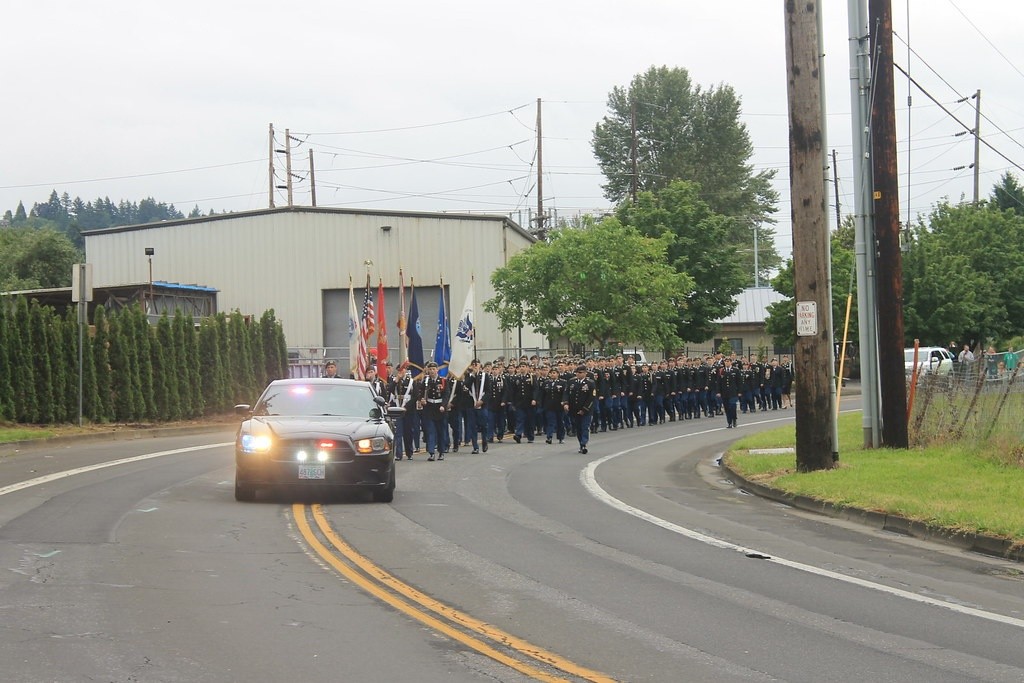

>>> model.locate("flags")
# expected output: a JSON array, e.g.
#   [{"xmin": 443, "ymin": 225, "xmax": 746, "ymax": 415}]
[
  {"xmin": 377, "ymin": 283, "xmax": 389, "ymax": 385},
  {"xmin": 405, "ymin": 285, "xmax": 424, "ymax": 379},
  {"xmin": 348, "ymin": 284, "xmax": 361, "ymax": 380},
  {"xmin": 396, "ymin": 268, "xmax": 409, "ymax": 372},
  {"xmin": 358, "ymin": 283, "xmax": 374, "ymax": 380},
  {"xmin": 434, "ymin": 287, "xmax": 452, "ymax": 378},
  {"xmin": 448, "ymin": 283, "xmax": 477, "ymax": 381}
]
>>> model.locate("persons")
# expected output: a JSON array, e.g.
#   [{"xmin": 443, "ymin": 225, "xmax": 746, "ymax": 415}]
[
  {"xmin": 349, "ymin": 350, "xmax": 795, "ymax": 462},
  {"xmin": 321, "ymin": 359, "xmax": 343, "ymax": 378},
  {"xmin": 945, "ymin": 341, "xmax": 1019, "ymax": 388}
]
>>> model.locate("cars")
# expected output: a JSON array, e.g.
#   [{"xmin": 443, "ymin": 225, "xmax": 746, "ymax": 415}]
[
  {"xmin": 234, "ymin": 378, "xmax": 396, "ymax": 502},
  {"xmin": 905, "ymin": 347, "xmax": 955, "ymax": 391}
]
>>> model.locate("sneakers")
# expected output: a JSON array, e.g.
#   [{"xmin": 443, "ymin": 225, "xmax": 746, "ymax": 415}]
[
  {"xmin": 453, "ymin": 448, "xmax": 459, "ymax": 452},
  {"xmin": 443, "ymin": 448, "xmax": 450, "ymax": 453},
  {"xmin": 395, "ymin": 457, "xmax": 403, "ymax": 461},
  {"xmin": 483, "ymin": 445, "xmax": 488, "ymax": 452},
  {"xmin": 464, "ymin": 442, "xmax": 471, "ymax": 446},
  {"xmin": 414, "ymin": 448, "xmax": 420, "ymax": 452},
  {"xmin": 437, "ymin": 453, "xmax": 444, "ymax": 460},
  {"xmin": 472, "ymin": 448, "xmax": 480, "ymax": 454},
  {"xmin": 407, "ymin": 455, "xmax": 414, "ymax": 460},
  {"xmin": 427, "ymin": 454, "xmax": 435, "ymax": 461},
  {"xmin": 434, "ymin": 444, "xmax": 438, "ymax": 448}
]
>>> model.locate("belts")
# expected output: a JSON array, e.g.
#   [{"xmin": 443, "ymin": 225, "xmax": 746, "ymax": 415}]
[{"xmin": 426, "ymin": 398, "xmax": 443, "ymax": 403}]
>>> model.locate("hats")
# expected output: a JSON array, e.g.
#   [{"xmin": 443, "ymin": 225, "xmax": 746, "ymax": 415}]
[
  {"xmin": 325, "ymin": 360, "xmax": 336, "ymax": 368},
  {"xmin": 428, "ymin": 362, "xmax": 439, "ymax": 367},
  {"xmin": 483, "ymin": 350, "xmax": 791, "ymax": 373},
  {"xmin": 365, "ymin": 365, "xmax": 376, "ymax": 371},
  {"xmin": 471, "ymin": 358, "xmax": 482, "ymax": 364},
  {"xmin": 386, "ymin": 361, "xmax": 393, "ymax": 367}
]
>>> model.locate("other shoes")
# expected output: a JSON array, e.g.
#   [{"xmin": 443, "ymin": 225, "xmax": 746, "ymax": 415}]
[
  {"xmin": 768, "ymin": 405, "xmax": 772, "ymax": 409},
  {"xmin": 527, "ymin": 439, "xmax": 534, "ymax": 443},
  {"xmin": 778, "ymin": 404, "xmax": 782, "ymax": 408},
  {"xmin": 569, "ymin": 432, "xmax": 576, "ymax": 437},
  {"xmin": 493, "ymin": 432, "xmax": 498, "ymax": 437},
  {"xmin": 726, "ymin": 423, "xmax": 732, "ymax": 428},
  {"xmin": 513, "ymin": 434, "xmax": 521, "ymax": 443},
  {"xmin": 523, "ymin": 434, "xmax": 526, "ymax": 438},
  {"xmin": 773, "ymin": 406, "xmax": 777, "ymax": 410},
  {"xmin": 578, "ymin": 446, "xmax": 588, "ymax": 454},
  {"xmin": 486, "ymin": 439, "xmax": 493, "ymax": 443},
  {"xmin": 567, "ymin": 430, "xmax": 572, "ymax": 435},
  {"xmin": 546, "ymin": 437, "xmax": 552, "ymax": 444},
  {"xmin": 589, "ymin": 409, "xmax": 725, "ymax": 434},
  {"xmin": 508, "ymin": 430, "xmax": 515, "ymax": 434},
  {"xmin": 761, "ymin": 407, "xmax": 767, "ymax": 411},
  {"xmin": 750, "ymin": 407, "xmax": 757, "ymax": 412},
  {"xmin": 497, "ymin": 439, "xmax": 503, "ymax": 443},
  {"xmin": 733, "ymin": 422, "xmax": 737, "ymax": 427},
  {"xmin": 790, "ymin": 401, "xmax": 792, "ymax": 407},
  {"xmin": 557, "ymin": 439, "xmax": 565, "ymax": 444},
  {"xmin": 759, "ymin": 405, "xmax": 762, "ymax": 408},
  {"xmin": 742, "ymin": 410, "xmax": 746, "ymax": 413},
  {"xmin": 536, "ymin": 432, "xmax": 542, "ymax": 435},
  {"xmin": 781, "ymin": 406, "xmax": 788, "ymax": 409}
]
